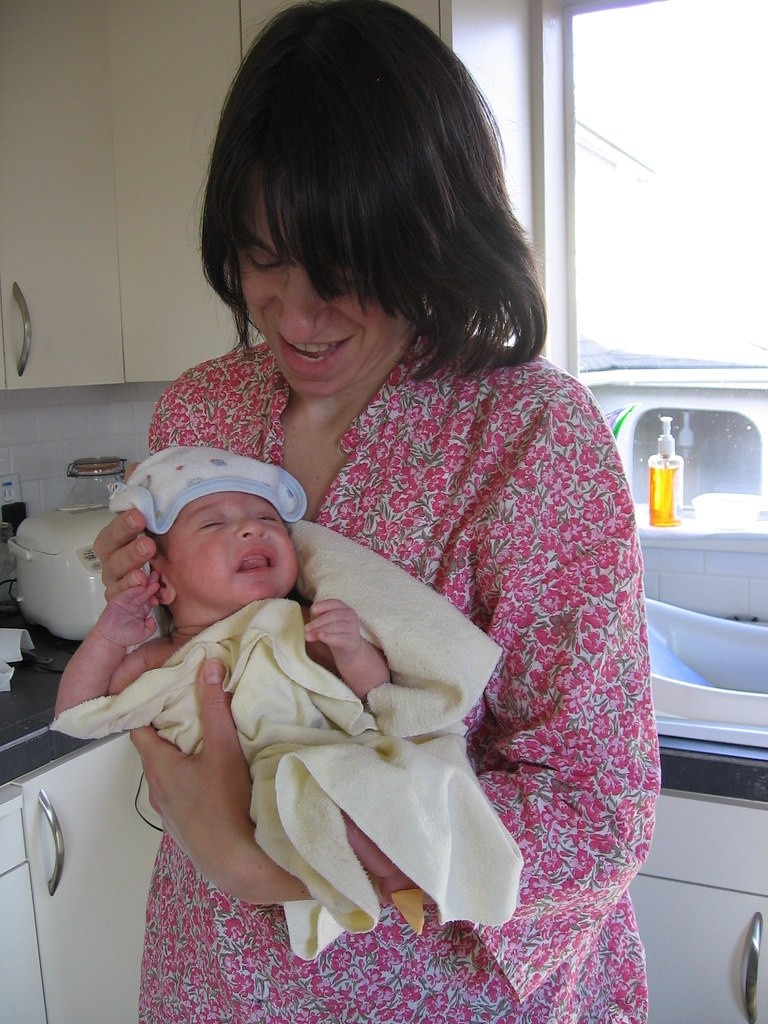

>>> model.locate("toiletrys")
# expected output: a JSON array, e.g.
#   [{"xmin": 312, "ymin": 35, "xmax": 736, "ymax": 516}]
[{"xmin": 648, "ymin": 417, "xmax": 682, "ymax": 526}]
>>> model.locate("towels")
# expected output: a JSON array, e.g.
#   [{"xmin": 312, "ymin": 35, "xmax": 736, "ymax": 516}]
[{"xmin": 48, "ymin": 520, "xmax": 524, "ymax": 961}]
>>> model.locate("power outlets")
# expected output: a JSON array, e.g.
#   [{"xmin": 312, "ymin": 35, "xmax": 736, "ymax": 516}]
[{"xmin": 0, "ymin": 473, "xmax": 22, "ymax": 539}]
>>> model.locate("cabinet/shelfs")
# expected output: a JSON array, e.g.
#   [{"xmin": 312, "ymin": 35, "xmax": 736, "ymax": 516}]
[
  {"xmin": 632, "ymin": 789, "xmax": 768, "ymax": 1024},
  {"xmin": 0, "ymin": 728, "xmax": 165, "ymax": 1024},
  {"xmin": 1, "ymin": 1, "xmax": 455, "ymax": 392}
]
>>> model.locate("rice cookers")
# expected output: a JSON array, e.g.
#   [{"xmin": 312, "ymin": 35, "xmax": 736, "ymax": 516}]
[{"xmin": 8, "ymin": 503, "xmax": 153, "ymax": 640}]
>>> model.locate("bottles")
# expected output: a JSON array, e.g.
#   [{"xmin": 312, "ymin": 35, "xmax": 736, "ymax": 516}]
[{"xmin": 67, "ymin": 456, "xmax": 126, "ymax": 504}]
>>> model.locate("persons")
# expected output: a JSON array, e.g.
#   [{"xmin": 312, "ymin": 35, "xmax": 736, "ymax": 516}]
[
  {"xmin": 53, "ymin": 445, "xmax": 420, "ymax": 909},
  {"xmin": 91, "ymin": 0, "xmax": 662, "ymax": 1024}
]
